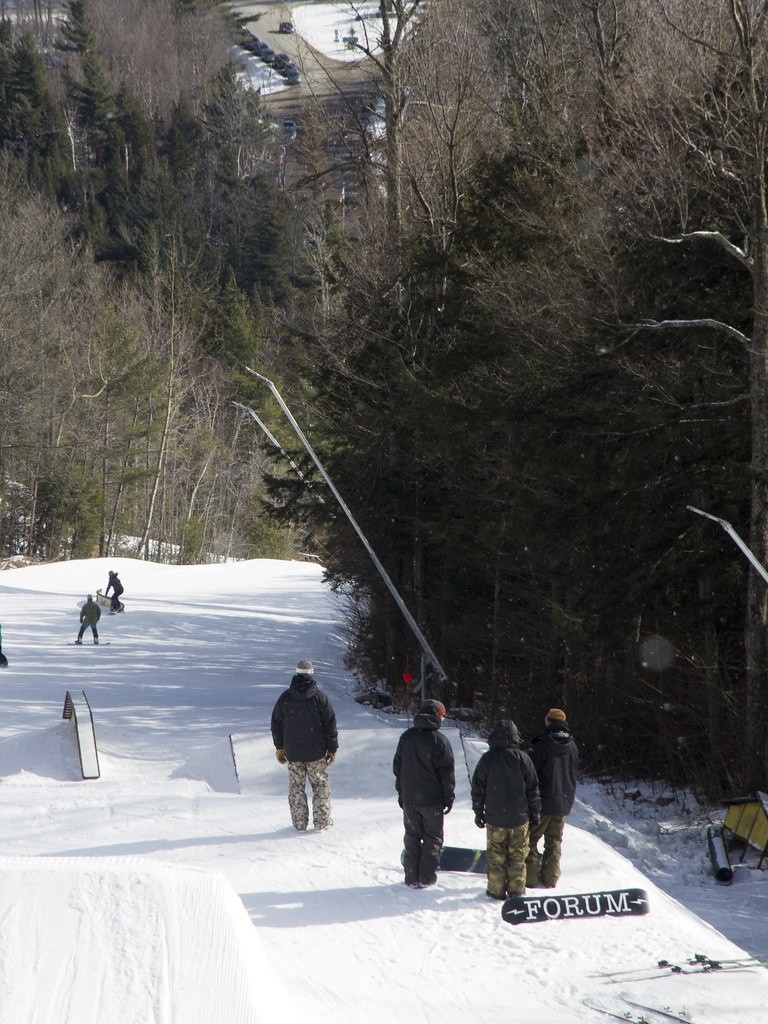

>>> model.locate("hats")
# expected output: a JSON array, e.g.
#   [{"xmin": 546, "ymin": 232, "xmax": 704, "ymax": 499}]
[
  {"xmin": 297, "ymin": 659, "xmax": 314, "ymax": 674},
  {"xmin": 421, "ymin": 699, "xmax": 446, "ymax": 718},
  {"xmin": 544, "ymin": 708, "xmax": 566, "ymax": 724},
  {"xmin": 109, "ymin": 572, "xmax": 114, "ymax": 576},
  {"xmin": 87, "ymin": 594, "xmax": 93, "ymax": 600}
]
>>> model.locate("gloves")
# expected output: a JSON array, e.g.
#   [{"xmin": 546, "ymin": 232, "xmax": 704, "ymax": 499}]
[
  {"xmin": 531, "ymin": 813, "xmax": 541, "ymax": 825},
  {"xmin": 398, "ymin": 798, "xmax": 403, "ymax": 809},
  {"xmin": 276, "ymin": 749, "xmax": 287, "ymax": 764},
  {"xmin": 326, "ymin": 751, "xmax": 335, "ymax": 765},
  {"xmin": 475, "ymin": 813, "xmax": 486, "ymax": 828},
  {"xmin": 442, "ymin": 801, "xmax": 452, "ymax": 815}
]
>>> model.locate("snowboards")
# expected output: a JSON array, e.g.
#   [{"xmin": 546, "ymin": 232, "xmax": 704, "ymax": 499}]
[
  {"xmin": 110, "ymin": 609, "xmax": 119, "ymax": 614},
  {"xmin": 500, "ymin": 888, "xmax": 649, "ymax": 925},
  {"xmin": 68, "ymin": 642, "xmax": 110, "ymax": 645},
  {"xmin": 400, "ymin": 844, "xmax": 543, "ymax": 875}
]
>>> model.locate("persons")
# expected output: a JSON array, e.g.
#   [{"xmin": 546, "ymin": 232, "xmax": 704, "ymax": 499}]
[
  {"xmin": 470, "ymin": 720, "xmax": 542, "ymax": 901},
  {"xmin": 0, "ymin": 623, "xmax": 8, "ymax": 667},
  {"xmin": 75, "ymin": 597, "xmax": 101, "ymax": 644},
  {"xmin": 271, "ymin": 660, "xmax": 339, "ymax": 832},
  {"xmin": 393, "ymin": 699, "xmax": 456, "ymax": 889},
  {"xmin": 525, "ymin": 708, "xmax": 579, "ymax": 888},
  {"xmin": 105, "ymin": 571, "xmax": 124, "ymax": 611}
]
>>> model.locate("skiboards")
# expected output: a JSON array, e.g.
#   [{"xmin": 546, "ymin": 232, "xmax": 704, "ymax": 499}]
[
  {"xmin": 593, "ymin": 954, "xmax": 767, "ymax": 986},
  {"xmin": 582, "ymin": 991, "xmax": 695, "ymax": 1023}
]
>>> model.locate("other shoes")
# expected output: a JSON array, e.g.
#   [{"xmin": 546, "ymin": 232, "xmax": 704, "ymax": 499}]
[
  {"xmin": 293, "ymin": 826, "xmax": 306, "ymax": 832},
  {"xmin": 316, "ymin": 819, "xmax": 333, "ymax": 832},
  {"xmin": 507, "ymin": 891, "xmax": 523, "ymax": 899},
  {"xmin": 486, "ymin": 889, "xmax": 506, "ymax": 900},
  {"xmin": 76, "ymin": 641, "xmax": 82, "ymax": 644},
  {"xmin": 419, "ymin": 874, "xmax": 437, "ymax": 888},
  {"xmin": 408, "ymin": 881, "xmax": 419, "ymax": 888},
  {"xmin": 538, "ymin": 876, "xmax": 555, "ymax": 888},
  {"xmin": 526, "ymin": 884, "xmax": 539, "ymax": 888}
]
